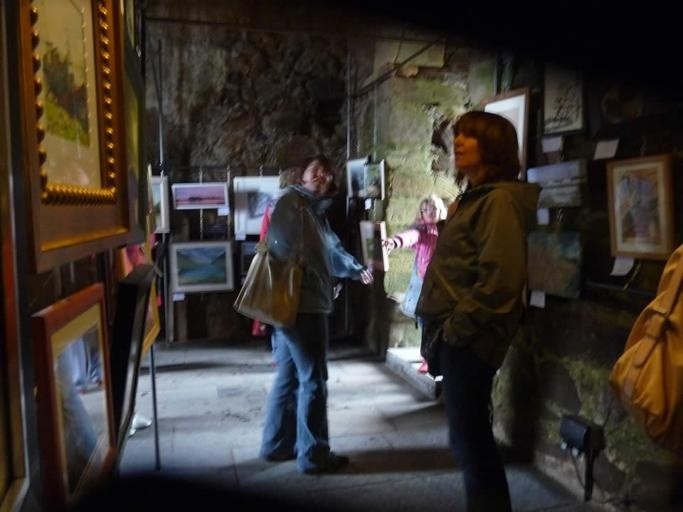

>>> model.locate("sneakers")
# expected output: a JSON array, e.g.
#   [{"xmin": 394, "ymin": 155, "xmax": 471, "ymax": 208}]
[{"xmin": 324, "ymin": 456, "xmax": 350, "ymax": 476}]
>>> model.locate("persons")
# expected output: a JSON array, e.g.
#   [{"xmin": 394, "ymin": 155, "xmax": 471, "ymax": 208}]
[
  {"xmin": 379, "ymin": 195, "xmax": 450, "ymax": 374},
  {"xmin": 413, "ymin": 107, "xmax": 543, "ymax": 511},
  {"xmin": 256, "ymin": 162, "xmax": 372, "ymax": 386},
  {"xmin": 259, "ymin": 156, "xmax": 350, "ymax": 476}
]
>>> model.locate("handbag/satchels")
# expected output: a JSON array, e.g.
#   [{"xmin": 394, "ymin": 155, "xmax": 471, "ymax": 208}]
[
  {"xmin": 231, "ymin": 250, "xmax": 303, "ymax": 329},
  {"xmin": 400, "ymin": 277, "xmax": 423, "ymax": 319}
]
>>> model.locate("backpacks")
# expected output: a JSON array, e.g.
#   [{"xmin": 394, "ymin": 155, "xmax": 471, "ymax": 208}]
[{"xmin": 609, "ymin": 244, "xmax": 682, "ymax": 457}]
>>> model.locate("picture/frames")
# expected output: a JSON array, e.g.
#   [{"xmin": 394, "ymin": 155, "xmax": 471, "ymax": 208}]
[
  {"xmin": 5, "ymin": 0, "xmax": 148, "ymax": 274},
  {"xmin": 111, "ymin": 265, "xmax": 154, "ymax": 454},
  {"xmin": 363, "ymin": 159, "xmax": 386, "ymax": 201},
  {"xmin": 605, "ymin": 154, "xmax": 674, "ymax": 261},
  {"xmin": 345, "ymin": 155, "xmax": 369, "ymax": 198},
  {"xmin": 360, "ymin": 220, "xmax": 390, "ymax": 271},
  {"xmin": 169, "ymin": 240, "xmax": 234, "ymax": 293},
  {"xmin": 151, "ymin": 176, "xmax": 288, "ymax": 241},
  {"xmin": 480, "ymin": 51, "xmax": 590, "ymax": 182},
  {"xmin": 32, "ymin": 283, "xmax": 120, "ymax": 512}
]
